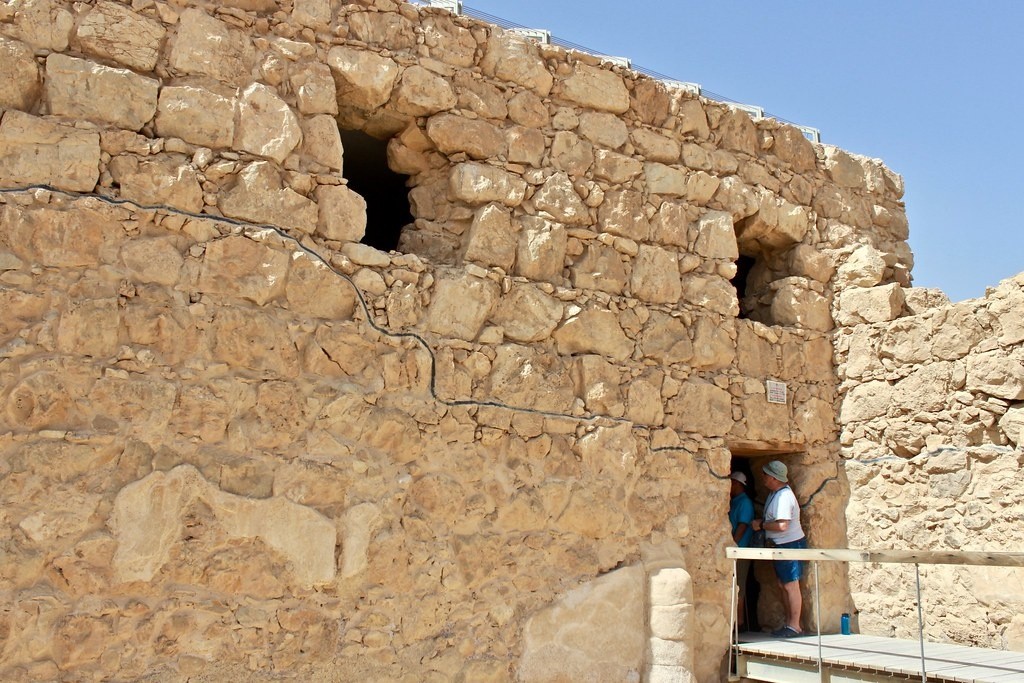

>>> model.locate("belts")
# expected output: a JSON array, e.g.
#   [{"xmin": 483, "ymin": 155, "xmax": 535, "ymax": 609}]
[{"xmin": 794, "ymin": 537, "xmax": 804, "ymax": 542}]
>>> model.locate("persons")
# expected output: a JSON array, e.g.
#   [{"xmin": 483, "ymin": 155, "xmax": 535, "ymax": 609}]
[
  {"xmin": 750, "ymin": 461, "xmax": 808, "ymax": 638},
  {"xmin": 728, "ymin": 472, "xmax": 755, "ymax": 632}
]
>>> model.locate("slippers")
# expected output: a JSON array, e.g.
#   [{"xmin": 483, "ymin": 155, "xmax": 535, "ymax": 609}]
[{"xmin": 770, "ymin": 626, "xmax": 800, "ymax": 637}]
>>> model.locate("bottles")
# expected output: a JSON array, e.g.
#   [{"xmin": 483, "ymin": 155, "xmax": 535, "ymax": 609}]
[{"xmin": 841, "ymin": 613, "xmax": 850, "ymax": 635}]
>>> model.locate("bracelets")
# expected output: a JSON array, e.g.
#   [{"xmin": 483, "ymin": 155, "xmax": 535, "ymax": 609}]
[{"xmin": 759, "ymin": 521, "xmax": 764, "ymax": 530}]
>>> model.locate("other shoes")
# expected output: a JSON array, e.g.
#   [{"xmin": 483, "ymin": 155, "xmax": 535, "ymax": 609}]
[{"xmin": 734, "ymin": 623, "xmax": 745, "ymax": 632}]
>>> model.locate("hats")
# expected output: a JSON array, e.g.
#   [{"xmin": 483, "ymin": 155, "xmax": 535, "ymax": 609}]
[
  {"xmin": 762, "ymin": 461, "xmax": 788, "ymax": 482},
  {"xmin": 731, "ymin": 472, "xmax": 747, "ymax": 486}
]
